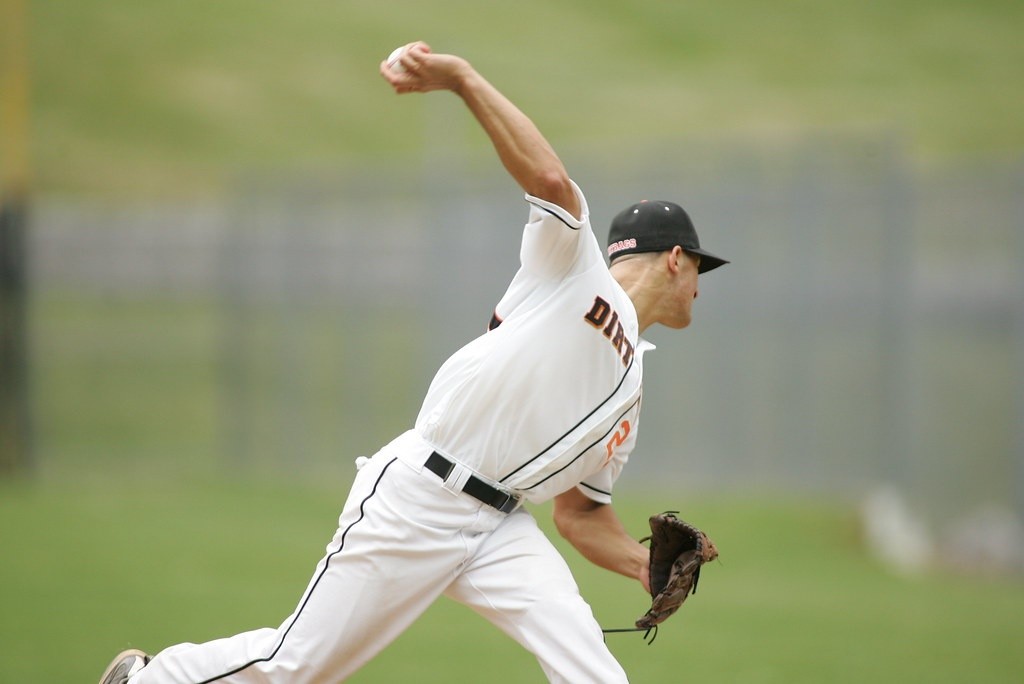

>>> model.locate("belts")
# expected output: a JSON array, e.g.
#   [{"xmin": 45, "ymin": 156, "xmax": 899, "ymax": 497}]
[{"xmin": 424, "ymin": 451, "xmax": 519, "ymax": 514}]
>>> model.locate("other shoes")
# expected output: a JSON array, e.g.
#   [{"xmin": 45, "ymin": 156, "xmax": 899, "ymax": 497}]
[{"xmin": 99, "ymin": 648, "xmax": 153, "ymax": 684}]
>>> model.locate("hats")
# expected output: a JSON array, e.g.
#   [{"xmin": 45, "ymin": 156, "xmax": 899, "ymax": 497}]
[{"xmin": 608, "ymin": 200, "xmax": 731, "ymax": 274}]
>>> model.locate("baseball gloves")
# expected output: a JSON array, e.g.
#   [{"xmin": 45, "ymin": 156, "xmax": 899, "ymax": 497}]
[{"xmin": 632, "ymin": 512, "xmax": 720, "ymax": 635}]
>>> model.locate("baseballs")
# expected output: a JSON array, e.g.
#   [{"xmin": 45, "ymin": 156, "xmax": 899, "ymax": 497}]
[{"xmin": 386, "ymin": 47, "xmax": 415, "ymax": 78}]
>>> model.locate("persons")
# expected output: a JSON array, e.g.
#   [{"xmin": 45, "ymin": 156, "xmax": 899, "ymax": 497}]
[{"xmin": 98, "ymin": 40, "xmax": 732, "ymax": 684}]
